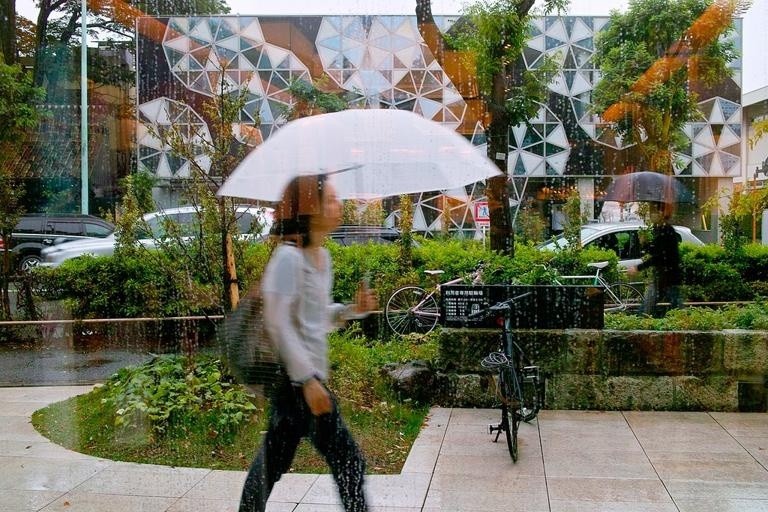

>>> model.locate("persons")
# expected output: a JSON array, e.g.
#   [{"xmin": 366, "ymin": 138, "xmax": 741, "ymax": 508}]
[
  {"xmin": 623, "ymin": 203, "xmax": 683, "ymax": 318},
  {"xmin": 551, "ymin": 206, "xmax": 571, "ymax": 234},
  {"xmin": 238, "ymin": 172, "xmax": 385, "ymax": 511}
]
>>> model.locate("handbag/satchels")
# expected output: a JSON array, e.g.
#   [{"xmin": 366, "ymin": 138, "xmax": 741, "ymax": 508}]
[{"xmin": 214, "ymin": 286, "xmax": 282, "ymax": 386}]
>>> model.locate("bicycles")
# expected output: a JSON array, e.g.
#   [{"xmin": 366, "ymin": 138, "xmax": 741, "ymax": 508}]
[
  {"xmin": 468, "ymin": 292, "xmax": 542, "ymax": 463},
  {"xmin": 537, "ymin": 255, "xmax": 645, "ymax": 316},
  {"xmin": 385, "ymin": 261, "xmax": 490, "ymax": 339}
]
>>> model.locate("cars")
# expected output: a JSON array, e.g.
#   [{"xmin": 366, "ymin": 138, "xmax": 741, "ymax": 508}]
[
  {"xmin": 329, "ymin": 224, "xmax": 422, "ymax": 276},
  {"xmin": 535, "ymin": 222, "xmax": 708, "ymax": 270},
  {"xmin": 40, "ymin": 204, "xmax": 276, "ymax": 271}
]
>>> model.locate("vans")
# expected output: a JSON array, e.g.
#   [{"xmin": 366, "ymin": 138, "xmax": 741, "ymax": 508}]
[{"xmin": 0, "ymin": 212, "xmax": 118, "ymax": 276}]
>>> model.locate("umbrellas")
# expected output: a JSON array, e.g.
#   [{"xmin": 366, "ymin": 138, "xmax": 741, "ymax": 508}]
[
  {"xmin": 214, "ymin": 106, "xmax": 507, "ymax": 204},
  {"xmin": 599, "ymin": 171, "xmax": 700, "ymax": 208}
]
